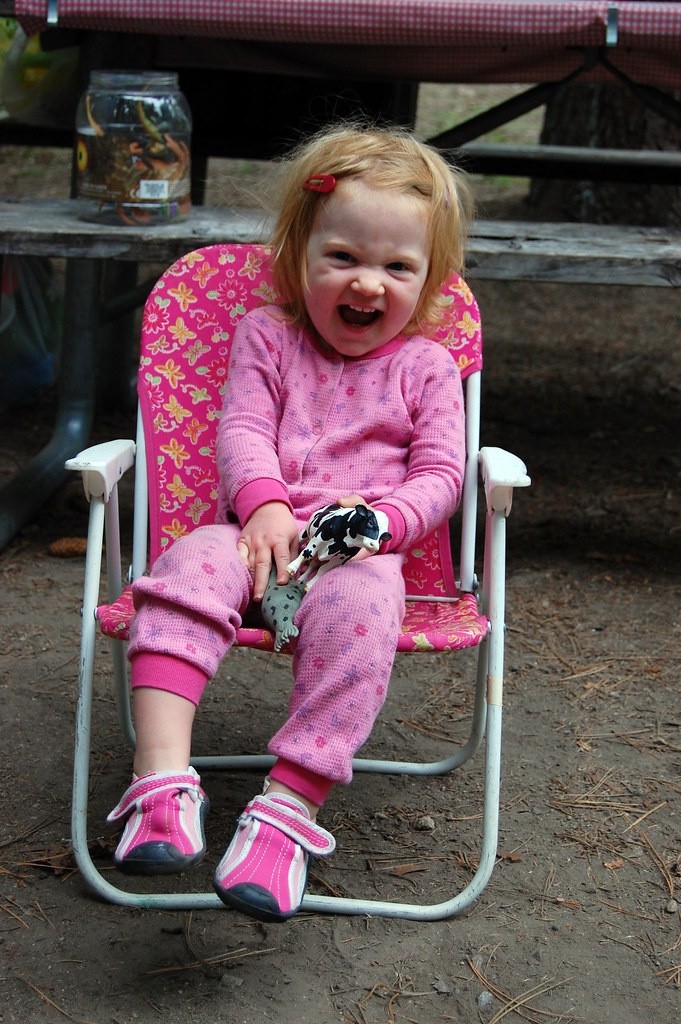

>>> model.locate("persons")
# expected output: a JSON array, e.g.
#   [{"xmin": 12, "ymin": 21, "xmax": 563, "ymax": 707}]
[{"xmin": 107, "ymin": 126, "xmax": 462, "ymax": 923}]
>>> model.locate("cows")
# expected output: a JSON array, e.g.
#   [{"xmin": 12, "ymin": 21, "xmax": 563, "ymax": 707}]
[{"xmin": 283, "ymin": 502, "xmax": 393, "ymax": 591}]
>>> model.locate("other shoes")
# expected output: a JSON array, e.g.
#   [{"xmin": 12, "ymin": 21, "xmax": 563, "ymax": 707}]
[
  {"xmin": 105, "ymin": 763, "xmax": 210, "ymax": 874},
  {"xmin": 211, "ymin": 775, "xmax": 337, "ymax": 923}
]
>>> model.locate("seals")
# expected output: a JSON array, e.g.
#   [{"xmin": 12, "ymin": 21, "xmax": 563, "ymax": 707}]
[{"xmin": 261, "ymin": 566, "xmax": 308, "ymax": 654}]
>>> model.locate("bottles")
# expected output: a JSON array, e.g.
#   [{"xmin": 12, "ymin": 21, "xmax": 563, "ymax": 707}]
[{"xmin": 74, "ymin": 68, "xmax": 194, "ymax": 225}]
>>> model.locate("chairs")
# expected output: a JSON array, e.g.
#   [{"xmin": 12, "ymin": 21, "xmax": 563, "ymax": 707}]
[{"xmin": 63, "ymin": 245, "xmax": 530, "ymax": 919}]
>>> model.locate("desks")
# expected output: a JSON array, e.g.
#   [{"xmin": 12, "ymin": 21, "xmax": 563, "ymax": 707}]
[{"xmin": 0, "ymin": 0, "xmax": 681, "ymax": 222}]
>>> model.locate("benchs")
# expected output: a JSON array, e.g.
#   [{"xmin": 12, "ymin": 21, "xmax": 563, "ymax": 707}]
[{"xmin": 0, "ymin": 145, "xmax": 681, "ymax": 550}]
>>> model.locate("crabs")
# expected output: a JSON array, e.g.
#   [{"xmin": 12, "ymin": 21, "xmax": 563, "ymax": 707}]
[{"xmin": 85, "ymin": 79, "xmax": 194, "ymax": 227}]
